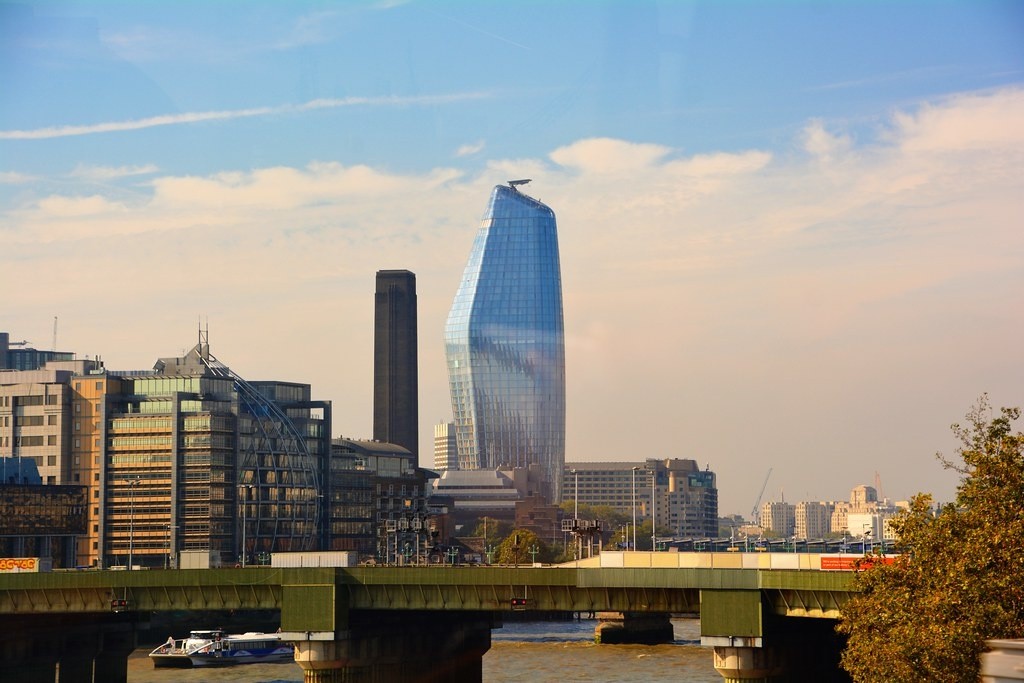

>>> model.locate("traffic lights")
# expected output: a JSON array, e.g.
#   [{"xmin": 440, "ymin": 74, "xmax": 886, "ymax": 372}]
[
  {"xmin": 511, "ymin": 598, "xmax": 527, "ymax": 605},
  {"xmin": 112, "ymin": 599, "xmax": 127, "ymax": 606}
]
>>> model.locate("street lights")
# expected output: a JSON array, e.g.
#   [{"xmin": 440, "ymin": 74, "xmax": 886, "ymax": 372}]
[
  {"xmin": 728, "ymin": 524, "xmax": 874, "ymax": 553},
  {"xmin": 164, "ymin": 523, "xmax": 171, "ymax": 569},
  {"xmin": 633, "ymin": 466, "xmax": 641, "ymax": 550},
  {"xmin": 243, "ymin": 483, "xmax": 255, "ymax": 567},
  {"xmin": 130, "ymin": 477, "xmax": 140, "ymax": 570},
  {"xmin": 571, "ymin": 469, "xmax": 577, "ymax": 559}
]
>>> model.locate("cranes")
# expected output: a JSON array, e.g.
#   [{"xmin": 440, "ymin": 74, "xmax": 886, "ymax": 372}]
[{"xmin": 751, "ymin": 468, "xmax": 772, "ymax": 524}]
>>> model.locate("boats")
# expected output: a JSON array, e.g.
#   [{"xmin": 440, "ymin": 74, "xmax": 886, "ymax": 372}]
[{"xmin": 147, "ymin": 629, "xmax": 296, "ymax": 668}]
